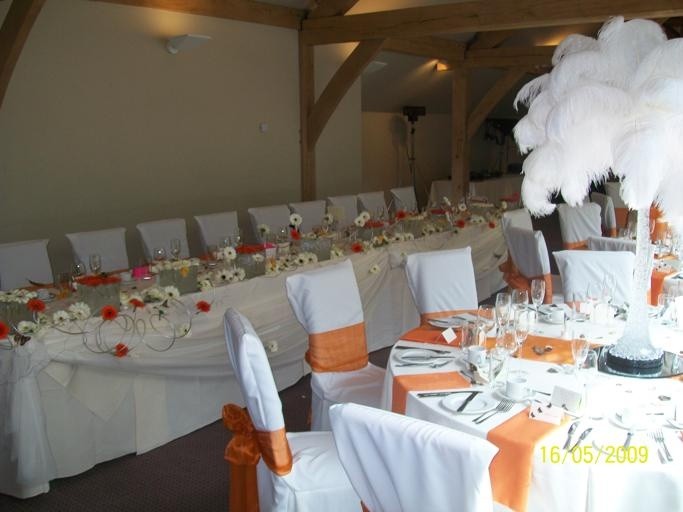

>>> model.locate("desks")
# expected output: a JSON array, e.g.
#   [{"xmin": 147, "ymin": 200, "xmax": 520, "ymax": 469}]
[{"xmin": 7, "ymin": 211, "xmax": 509, "ymax": 497}]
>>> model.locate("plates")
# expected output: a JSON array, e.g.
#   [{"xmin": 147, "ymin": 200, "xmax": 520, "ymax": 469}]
[
  {"xmin": 393, "ymin": 348, "xmax": 440, "ymax": 364},
  {"xmin": 442, "ymin": 391, "xmax": 497, "ymax": 414},
  {"xmin": 428, "ymin": 315, "xmax": 468, "ymax": 328},
  {"xmin": 592, "ymin": 432, "xmax": 658, "ymax": 460},
  {"xmin": 33, "ymin": 288, "xmax": 61, "ymax": 302}
]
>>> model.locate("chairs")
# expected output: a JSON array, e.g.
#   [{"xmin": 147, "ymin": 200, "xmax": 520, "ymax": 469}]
[
  {"xmin": 584, "ymin": 448, "xmax": 680, "ymax": 512},
  {"xmin": 328, "ymin": 402, "xmax": 496, "ymax": 510},
  {"xmin": 1, "ymin": 180, "xmax": 521, "ymax": 299},
  {"xmin": 219, "ymin": 307, "xmax": 365, "ymax": 511}
]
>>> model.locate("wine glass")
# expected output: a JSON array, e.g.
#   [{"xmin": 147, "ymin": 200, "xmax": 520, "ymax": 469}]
[
  {"xmin": 463, "ymin": 275, "xmax": 683, "ymax": 431},
  {"xmin": 69, "ymin": 224, "xmax": 292, "ymax": 285},
  {"xmin": 617, "ymin": 214, "xmax": 683, "ymax": 273}
]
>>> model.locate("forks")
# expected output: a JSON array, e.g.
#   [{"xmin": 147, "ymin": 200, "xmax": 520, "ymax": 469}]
[{"xmin": 647, "ymin": 428, "xmax": 674, "ymax": 464}]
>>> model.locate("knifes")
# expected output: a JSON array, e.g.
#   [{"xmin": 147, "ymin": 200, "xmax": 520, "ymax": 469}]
[{"xmin": 563, "ymin": 422, "xmax": 592, "ymax": 455}]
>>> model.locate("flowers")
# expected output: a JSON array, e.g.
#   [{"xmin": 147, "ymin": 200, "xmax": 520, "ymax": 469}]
[{"xmin": 1, "ymin": 301, "xmax": 214, "ymax": 361}]
